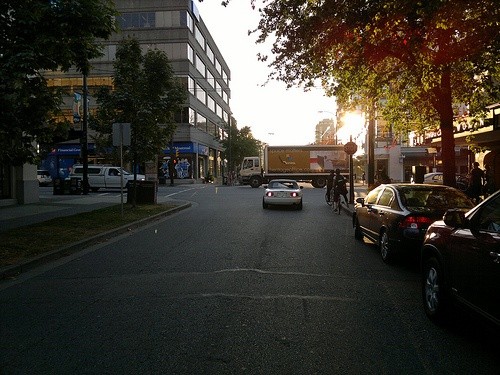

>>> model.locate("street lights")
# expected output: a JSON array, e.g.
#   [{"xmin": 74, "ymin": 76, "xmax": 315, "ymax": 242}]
[{"xmin": 318, "ymin": 110, "xmax": 337, "ymax": 145}]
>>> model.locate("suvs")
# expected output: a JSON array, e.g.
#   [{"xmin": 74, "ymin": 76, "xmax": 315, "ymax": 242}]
[{"xmin": 69, "ymin": 164, "xmax": 145, "ymax": 191}]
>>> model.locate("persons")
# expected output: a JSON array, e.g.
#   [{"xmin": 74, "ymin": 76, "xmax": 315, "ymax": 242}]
[
  {"xmin": 231, "ymin": 171, "xmax": 237, "ymax": 184},
  {"xmin": 469, "ymin": 162, "xmax": 485, "ymax": 204},
  {"xmin": 326, "ymin": 169, "xmax": 349, "ymax": 212}
]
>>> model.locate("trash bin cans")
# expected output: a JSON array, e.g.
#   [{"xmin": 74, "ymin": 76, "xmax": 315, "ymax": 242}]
[
  {"xmin": 53, "ymin": 177, "xmax": 66, "ymax": 194},
  {"xmin": 126, "ymin": 179, "xmax": 158, "ymax": 204},
  {"xmin": 159, "ymin": 176, "xmax": 166, "ymax": 184},
  {"xmin": 70, "ymin": 177, "xmax": 82, "ymax": 195}
]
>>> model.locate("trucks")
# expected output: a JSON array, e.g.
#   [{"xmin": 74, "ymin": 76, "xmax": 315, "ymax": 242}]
[{"xmin": 239, "ymin": 145, "xmax": 350, "ymax": 188}]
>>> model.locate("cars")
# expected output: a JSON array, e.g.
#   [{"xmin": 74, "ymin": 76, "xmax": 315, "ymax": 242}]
[
  {"xmin": 263, "ymin": 179, "xmax": 304, "ymax": 210},
  {"xmin": 353, "ymin": 184, "xmax": 500, "ymax": 318},
  {"xmin": 411, "ymin": 172, "xmax": 470, "ymax": 188},
  {"xmin": 37, "ymin": 170, "xmax": 52, "ymax": 187}
]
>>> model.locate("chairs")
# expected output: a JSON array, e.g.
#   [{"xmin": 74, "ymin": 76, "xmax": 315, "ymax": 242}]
[{"xmin": 408, "ymin": 198, "xmax": 420, "ymax": 207}]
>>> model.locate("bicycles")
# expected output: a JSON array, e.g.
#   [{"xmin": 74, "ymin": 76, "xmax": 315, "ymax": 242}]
[{"xmin": 325, "ymin": 186, "xmax": 341, "ymax": 215}]
